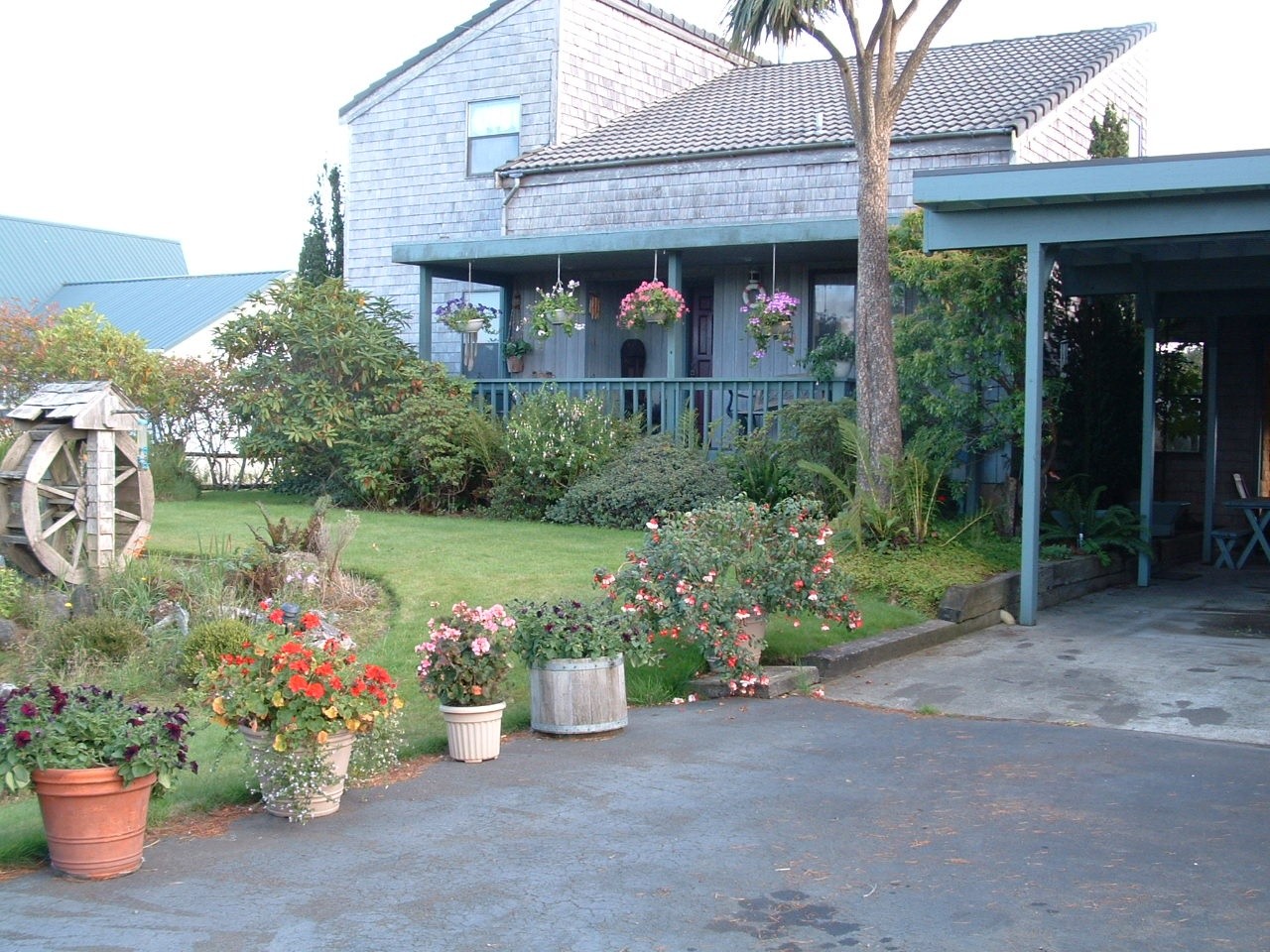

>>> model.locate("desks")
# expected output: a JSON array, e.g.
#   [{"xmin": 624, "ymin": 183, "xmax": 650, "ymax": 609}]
[{"xmin": 1220, "ymin": 496, "xmax": 1270, "ymax": 568}]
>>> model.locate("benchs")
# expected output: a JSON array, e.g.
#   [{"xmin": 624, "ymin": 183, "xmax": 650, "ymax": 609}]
[{"xmin": 1210, "ymin": 526, "xmax": 1254, "ymax": 569}]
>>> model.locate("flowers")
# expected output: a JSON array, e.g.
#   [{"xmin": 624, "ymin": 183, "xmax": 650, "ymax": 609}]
[
  {"xmin": 618, "ymin": 280, "xmax": 689, "ymax": 330},
  {"xmin": 504, "ymin": 596, "xmax": 643, "ymax": 735},
  {"xmin": 434, "ymin": 297, "xmax": 495, "ymax": 333},
  {"xmin": 606, "ymin": 492, "xmax": 846, "ymax": 682},
  {"xmin": 3, "ymin": 681, "xmax": 204, "ymax": 880},
  {"xmin": 209, "ymin": 602, "xmax": 398, "ymax": 821},
  {"xmin": 516, "ymin": 280, "xmax": 585, "ymax": 339},
  {"xmin": 739, "ymin": 289, "xmax": 794, "ymax": 359},
  {"xmin": 415, "ymin": 594, "xmax": 523, "ymax": 761}
]
